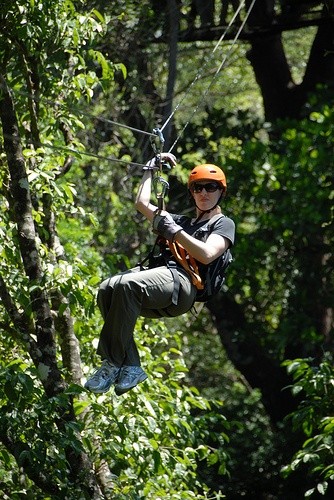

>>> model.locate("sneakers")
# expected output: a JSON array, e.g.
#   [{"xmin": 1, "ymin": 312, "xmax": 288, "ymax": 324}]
[
  {"xmin": 115, "ymin": 365, "xmax": 148, "ymax": 396},
  {"xmin": 84, "ymin": 360, "xmax": 121, "ymax": 394}
]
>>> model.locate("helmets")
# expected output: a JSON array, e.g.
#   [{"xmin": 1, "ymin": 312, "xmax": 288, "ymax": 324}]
[{"xmin": 189, "ymin": 164, "xmax": 227, "ymax": 191}]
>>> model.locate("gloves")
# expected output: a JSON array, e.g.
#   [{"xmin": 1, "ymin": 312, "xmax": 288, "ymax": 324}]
[
  {"xmin": 152, "ymin": 207, "xmax": 183, "ymax": 243},
  {"xmin": 142, "ymin": 152, "xmax": 177, "ymax": 178}
]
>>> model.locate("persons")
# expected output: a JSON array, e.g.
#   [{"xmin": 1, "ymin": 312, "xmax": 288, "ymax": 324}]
[{"xmin": 84, "ymin": 152, "xmax": 236, "ymax": 395}]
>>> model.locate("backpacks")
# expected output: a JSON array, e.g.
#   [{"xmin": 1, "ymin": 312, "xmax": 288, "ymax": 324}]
[{"xmin": 200, "ymin": 215, "xmax": 232, "ymax": 302}]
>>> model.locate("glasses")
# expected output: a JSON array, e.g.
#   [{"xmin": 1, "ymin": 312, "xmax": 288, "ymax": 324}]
[{"xmin": 191, "ymin": 182, "xmax": 222, "ymax": 193}]
